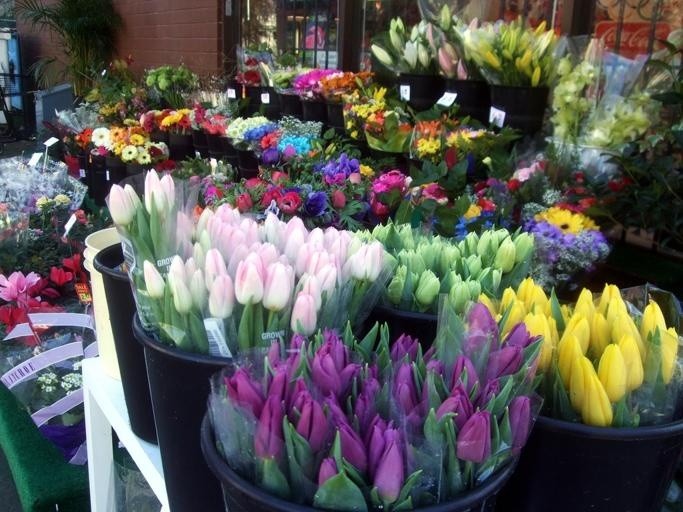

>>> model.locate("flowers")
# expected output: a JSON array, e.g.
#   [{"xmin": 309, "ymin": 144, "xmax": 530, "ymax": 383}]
[
  {"xmin": 85, "ymin": 63, "xmax": 230, "ymax": 119},
  {"xmin": 197, "ymin": 177, "xmax": 330, "ymax": 229},
  {"xmin": 354, "ymin": 217, "xmax": 534, "ymax": 314},
  {"xmin": 223, "ymin": 303, "xmax": 545, "ymax": 508},
  {"xmin": 505, "ymin": 30, "xmax": 683, "ymax": 220},
  {"xmin": 370, "ymin": 3, "xmax": 559, "ymax": 88},
  {"xmin": 65, "ymin": 120, "xmax": 169, "ymax": 167},
  {"xmin": 0, "ymin": 194, "xmax": 98, "ymax": 466},
  {"xmin": 478, "ymin": 278, "xmax": 679, "ymax": 427},
  {"xmin": 234, "ymin": 49, "xmax": 489, "ymax": 162},
  {"xmin": 139, "ymin": 107, "xmax": 610, "ymax": 294},
  {"xmin": 109, "ymin": 168, "xmax": 386, "ymax": 358}
]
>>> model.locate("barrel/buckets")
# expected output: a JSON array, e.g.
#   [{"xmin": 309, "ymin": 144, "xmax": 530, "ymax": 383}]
[
  {"xmin": 201, "ymin": 411, "xmax": 521, "ymax": 511},
  {"xmin": 77, "ymin": 155, "xmax": 88, "ymax": 185},
  {"xmin": 167, "ymin": 134, "xmax": 191, "ymax": 161},
  {"xmin": 217, "ymin": 136, "xmax": 238, "ymax": 181},
  {"xmin": 236, "ymin": 147, "xmax": 259, "ymax": 179},
  {"xmin": 327, "ymin": 103, "xmax": 345, "ymax": 132},
  {"xmin": 104, "ymin": 156, "xmax": 126, "ymax": 207},
  {"xmin": 90, "ymin": 152, "xmax": 106, "ymax": 206},
  {"xmin": 489, "ymin": 84, "xmax": 550, "ymax": 140},
  {"xmin": 93, "ymin": 242, "xmax": 159, "ymax": 446},
  {"xmin": 133, "ymin": 310, "xmax": 234, "ymax": 511},
  {"xmin": 280, "ymin": 93, "xmax": 300, "ymax": 117},
  {"xmin": 203, "ymin": 132, "xmax": 224, "ymax": 162},
  {"xmin": 535, "ymin": 415, "xmax": 683, "ymax": 511},
  {"xmin": 260, "ymin": 88, "xmax": 280, "ymax": 113},
  {"xmin": 241, "ymin": 86, "xmax": 267, "ymax": 114},
  {"xmin": 189, "ymin": 125, "xmax": 208, "ymax": 158},
  {"xmin": 84, "ymin": 226, "xmax": 123, "ymax": 383},
  {"xmin": 445, "ymin": 78, "xmax": 489, "ymax": 128},
  {"xmin": 367, "ymin": 144, "xmax": 410, "ymax": 175},
  {"xmin": 63, "ymin": 152, "xmax": 84, "ymax": 183},
  {"xmin": 399, "ymin": 73, "xmax": 444, "ymax": 113},
  {"xmin": 373, "ymin": 303, "xmax": 439, "ymax": 358},
  {"xmin": 302, "ymin": 100, "xmax": 326, "ymax": 124}
]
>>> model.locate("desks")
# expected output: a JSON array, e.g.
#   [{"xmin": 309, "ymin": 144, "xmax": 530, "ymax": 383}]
[{"xmin": 80, "ymin": 358, "xmax": 171, "ymax": 512}]
